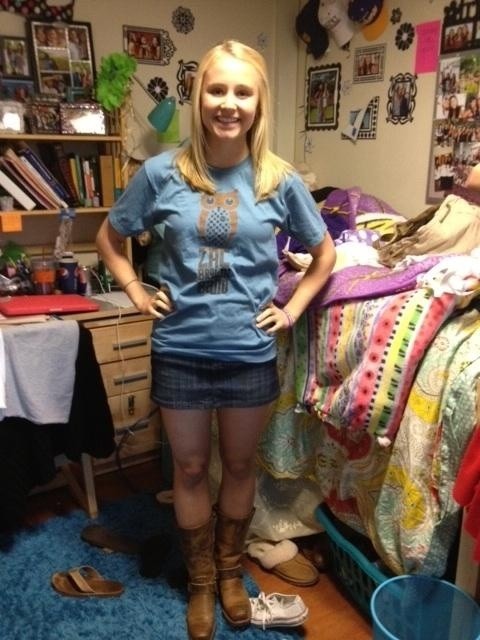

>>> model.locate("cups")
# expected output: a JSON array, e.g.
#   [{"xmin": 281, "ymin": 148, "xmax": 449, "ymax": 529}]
[
  {"xmin": 58, "ymin": 257, "xmax": 79, "ymax": 294},
  {"xmin": 31, "ymin": 257, "xmax": 55, "ymax": 295}
]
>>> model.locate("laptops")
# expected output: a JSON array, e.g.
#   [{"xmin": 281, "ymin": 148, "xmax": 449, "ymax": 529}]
[{"xmin": 0, "ymin": 294, "xmax": 99, "ymax": 316}]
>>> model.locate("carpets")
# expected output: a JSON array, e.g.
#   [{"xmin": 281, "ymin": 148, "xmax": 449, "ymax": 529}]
[{"xmin": 0, "ymin": 495, "xmax": 305, "ymax": 640}]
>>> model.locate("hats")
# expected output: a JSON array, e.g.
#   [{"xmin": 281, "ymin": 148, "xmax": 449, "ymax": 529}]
[
  {"xmin": 296, "ymin": 1, "xmax": 330, "ymax": 61},
  {"xmin": 318, "ymin": 0, "xmax": 354, "ymax": 49},
  {"xmin": 347, "ymin": 0, "xmax": 388, "ymax": 41}
]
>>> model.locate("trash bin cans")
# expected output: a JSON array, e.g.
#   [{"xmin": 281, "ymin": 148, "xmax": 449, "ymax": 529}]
[{"xmin": 370, "ymin": 575, "xmax": 480, "ymax": 640}]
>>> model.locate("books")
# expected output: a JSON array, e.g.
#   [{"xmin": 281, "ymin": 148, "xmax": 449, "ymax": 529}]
[{"xmin": 1, "ymin": 137, "xmax": 117, "ymax": 211}]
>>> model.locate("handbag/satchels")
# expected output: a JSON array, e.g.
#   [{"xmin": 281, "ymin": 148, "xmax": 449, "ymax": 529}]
[{"xmin": 379, "ymin": 195, "xmax": 479, "ymax": 269}]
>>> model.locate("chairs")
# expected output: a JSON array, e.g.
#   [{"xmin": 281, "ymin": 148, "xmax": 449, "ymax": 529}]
[{"xmin": 9, "ymin": 319, "xmax": 98, "ymax": 521}]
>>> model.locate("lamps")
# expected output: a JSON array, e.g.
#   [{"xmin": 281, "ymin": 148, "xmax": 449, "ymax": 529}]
[
  {"xmin": 133, "ymin": 72, "xmax": 176, "ymax": 132},
  {"xmin": 156, "ymin": 109, "xmax": 180, "ymax": 144}
]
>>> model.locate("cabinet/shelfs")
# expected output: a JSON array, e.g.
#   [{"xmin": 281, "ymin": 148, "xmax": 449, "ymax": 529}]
[
  {"xmin": 0, "ymin": 295, "xmax": 167, "ymax": 520},
  {"xmin": 0, "ymin": 99, "xmax": 133, "ymax": 294}
]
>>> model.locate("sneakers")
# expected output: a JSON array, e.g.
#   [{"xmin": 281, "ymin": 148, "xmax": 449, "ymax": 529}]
[{"xmin": 248, "ymin": 591, "xmax": 308, "ymax": 629}]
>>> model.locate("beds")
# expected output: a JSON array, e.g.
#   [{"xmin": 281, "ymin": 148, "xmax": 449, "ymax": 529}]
[{"xmin": 274, "ymin": 189, "xmax": 480, "ymax": 640}]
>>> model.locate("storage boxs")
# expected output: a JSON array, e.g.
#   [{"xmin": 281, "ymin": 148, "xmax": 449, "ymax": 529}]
[{"xmin": 313, "ymin": 500, "xmax": 404, "ymax": 625}]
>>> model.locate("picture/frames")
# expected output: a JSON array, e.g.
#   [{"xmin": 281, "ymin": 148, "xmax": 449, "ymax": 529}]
[
  {"xmin": 27, "ymin": 16, "xmax": 99, "ymax": 98},
  {"xmin": 30, "ymin": 101, "xmax": 62, "ymax": 134},
  {"xmin": 440, "ymin": 0, "xmax": 479, "ymax": 55},
  {"xmin": 340, "ymin": 96, "xmax": 379, "ymax": 143},
  {"xmin": 306, "ymin": 64, "xmax": 341, "ymax": 131},
  {"xmin": 385, "ymin": 71, "xmax": 417, "ymax": 125},
  {"xmin": 0, "ymin": 34, "xmax": 29, "ymax": 79},
  {"xmin": 121, "ymin": 22, "xmax": 176, "ymax": 66},
  {"xmin": 62, "ymin": 102, "xmax": 110, "ymax": 136},
  {"xmin": 425, "ymin": 49, "xmax": 480, "ymax": 206},
  {"xmin": 176, "ymin": 59, "xmax": 198, "ymax": 106},
  {"xmin": 1, "ymin": 80, "xmax": 35, "ymax": 103},
  {"xmin": 353, "ymin": 43, "xmax": 386, "ymax": 83}
]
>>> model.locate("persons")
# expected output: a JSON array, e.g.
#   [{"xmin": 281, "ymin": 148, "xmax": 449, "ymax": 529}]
[
  {"xmin": 7, "ymin": 42, "xmax": 17, "ymax": 74},
  {"xmin": 458, "ymin": 160, "xmax": 473, "ymax": 185},
  {"xmin": 128, "ymin": 32, "xmax": 137, "ymax": 56},
  {"xmin": 434, "ymin": 151, "xmax": 455, "ymax": 190},
  {"xmin": 446, "ymin": 29, "xmax": 455, "ymax": 47},
  {"xmin": 463, "ymin": 25, "xmax": 472, "ymax": 45},
  {"xmin": 463, "ymin": 96, "xmax": 480, "ymax": 119},
  {"xmin": 67, "ymin": 29, "xmax": 83, "ymax": 61},
  {"xmin": 359, "ymin": 54, "xmax": 378, "ymax": 74},
  {"xmin": 150, "ymin": 38, "xmax": 161, "ymax": 59},
  {"xmin": 80, "ymin": 70, "xmax": 90, "ymax": 86},
  {"xmin": 96, "ymin": 41, "xmax": 337, "ymax": 640},
  {"xmin": 448, "ymin": 96, "xmax": 462, "ymax": 120},
  {"xmin": 436, "ymin": 123, "xmax": 480, "ymax": 145},
  {"xmin": 314, "ymin": 81, "xmax": 330, "ymax": 122},
  {"xmin": 15, "ymin": 43, "xmax": 26, "ymax": 74},
  {"xmin": 38, "ymin": 115, "xmax": 53, "ymax": 130},
  {"xmin": 42, "ymin": 75, "xmax": 66, "ymax": 95},
  {"xmin": 35, "ymin": 26, "xmax": 65, "ymax": 49},
  {"xmin": 139, "ymin": 35, "xmax": 150, "ymax": 58},
  {"xmin": 437, "ymin": 93, "xmax": 449, "ymax": 118},
  {"xmin": 454, "ymin": 27, "xmax": 463, "ymax": 48},
  {"xmin": 438, "ymin": 71, "xmax": 457, "ymax": 93}
]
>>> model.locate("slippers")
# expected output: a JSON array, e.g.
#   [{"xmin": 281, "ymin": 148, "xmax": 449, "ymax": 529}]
[
  {"xmin": 249, "ymin": 539, "xmax": 319, "ymax": 587},
  {"xmin": 52, "ymin": 565, "xmax": 124, "ymax": 596},
  {"xmin": 80, "ymin": 523, "xmax": 141, "ymax": 556}
]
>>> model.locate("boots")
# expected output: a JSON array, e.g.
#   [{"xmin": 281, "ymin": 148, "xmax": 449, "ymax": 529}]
[
  {"xmin": 211, "ymin": 502, "xmax": 257, "ymax": 627},
  {"xmin": 170, "ymin": 510, "xmax": 217, "ymax": 640},
  {"xmin": 156, "ymin": 489, "xmax": 190, "ymax": 583}
]
